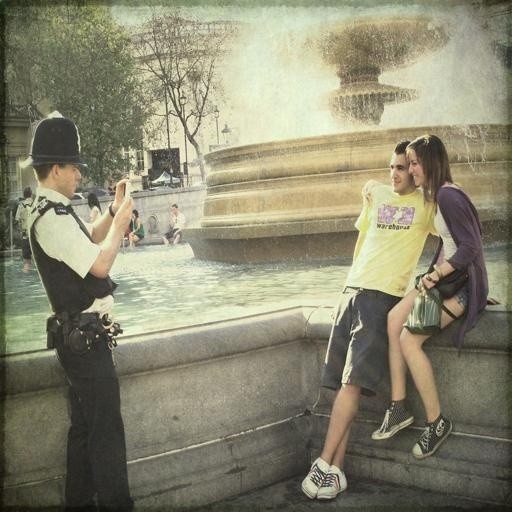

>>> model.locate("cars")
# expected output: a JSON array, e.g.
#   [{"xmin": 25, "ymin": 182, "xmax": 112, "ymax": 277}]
[{"xmin": 0, "ymin": 186, "xmax": 111, "ymax": 222}]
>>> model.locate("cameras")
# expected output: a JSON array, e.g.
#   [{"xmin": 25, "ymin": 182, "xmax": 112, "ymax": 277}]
[{"xmin": 123, "ymin": 182, "xmax": 130, "ymax": 197}]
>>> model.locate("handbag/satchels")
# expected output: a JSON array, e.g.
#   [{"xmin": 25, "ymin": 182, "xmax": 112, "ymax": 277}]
[
  {"xmin": 402, "ymin": 284, "xmax": 444, "ymax": 334},
  {"xmin": 433, "ymin": 266, "xmax": 468, "ymax": 300}
]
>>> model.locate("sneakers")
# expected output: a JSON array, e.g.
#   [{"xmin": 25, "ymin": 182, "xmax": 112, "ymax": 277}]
[
  {"xmin": 411, "ymin": 413, "xmax": 453, "ymax": 459},
  {"xmin": 370, "ymin": 397, "xmax": 415, "ymax": 441},
  {"xmin": 299, "ymin": 455, "xmax": 349, "ymax": 502}
]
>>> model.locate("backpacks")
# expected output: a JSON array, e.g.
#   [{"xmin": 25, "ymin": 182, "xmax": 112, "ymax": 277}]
[{"xmin": 18, "ymin": 201, "xmax": 31, "ymax": 230}]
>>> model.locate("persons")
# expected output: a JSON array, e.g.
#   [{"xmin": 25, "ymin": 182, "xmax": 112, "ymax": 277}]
[
  {"xmin": 128, "ymin": 210, "xmax": 144, "ymax": 250},
  {"xmin": 301, "ymin": 140, "xmax": 502, "ymax": 500},
  {"xmin": 14, "ymin": 188, "xmax": 37, "ymax": 273},
  {"xmin": 162, "ymin": 204, "xmax": 186, "ymax": 245},
  {"xmin": 27, "ymin": 119, "xmax": 136, "ymax": 512},
  {"xmin": 88, "ymin": 193, "xmax": 102, "ymax": 224},
  {"xmin": 362, "ymin": 133, "xmax": 489, "ymax": 461}
]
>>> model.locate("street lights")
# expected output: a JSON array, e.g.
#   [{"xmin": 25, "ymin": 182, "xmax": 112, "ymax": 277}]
[
  {"xmin": 212, "ymin": 103, "xmax": 222, "ymax": 147},
  {"xmin": 221, "ymin": 124, "xmax": 232, "ymax": 148},
  {"xmin": 180, "ymin": 91, "xmax": 191, "ymax": 186}
]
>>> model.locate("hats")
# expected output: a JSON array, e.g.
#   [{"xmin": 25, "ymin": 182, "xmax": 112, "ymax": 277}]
[{"xmin": 27, "ymin": 117, "xmax": 89, "ymax": 170}]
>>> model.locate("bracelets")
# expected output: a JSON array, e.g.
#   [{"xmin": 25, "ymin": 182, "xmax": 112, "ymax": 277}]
[
  {"xmin": 424, "ymin": 275, "xmax": 439, "ymax": 284},
  {"xmin": 108, "ymin": 202, "xmax": 116, "ymax": 218},
  {"xmin": 433, "ymin": 265, "xmax": 443, "ymax": 280}
]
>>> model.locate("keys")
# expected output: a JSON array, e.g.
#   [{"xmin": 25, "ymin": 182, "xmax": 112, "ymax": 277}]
[{"xmin": 100, "ymin": 313, "xmax": 123, "ymax": 350}]
